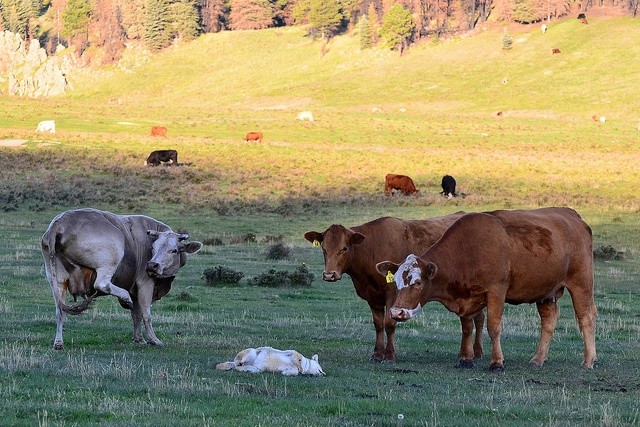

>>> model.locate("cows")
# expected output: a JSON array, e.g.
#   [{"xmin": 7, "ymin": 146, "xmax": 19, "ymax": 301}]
[
  {"xmin": 304, "ymin": 211, "xmax": 485, "ymax": 362},
  {"xmin": 246, "ymin": 132, "xmax": 263, "ymax": 142},
  {"xmin": 152, "ymin": 127, "xmax": 167, "ymax": 137},
  {"xmin": 441, "ymin": 175, "xmax": 456, "ymax": 200},
  {"xmin": 299, "ymin": 112, "xmax": 313, "ymax": 121},
  {"xmin": 147, "ymin": 151, "xmax": 177, "ymax": 167},
  {"xmin": 376, "ymin": 207, "xmax": 597, "ymax": 371},
  {"xmin": 41, "ymin": 208, "xmax": 202, "ymax": 351},
  {"xmin": 385, "ymin": 174, "xmax": 419, "ymax": 199},
  {"xmin": 38, "ymin": 121, "xmax": 55, "ymax": 133}
]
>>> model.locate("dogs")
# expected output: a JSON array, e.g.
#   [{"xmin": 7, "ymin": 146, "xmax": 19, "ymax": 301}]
[{"xmin": 215, "ymin": 348, "xmax": 326, "ymax": 377}]
